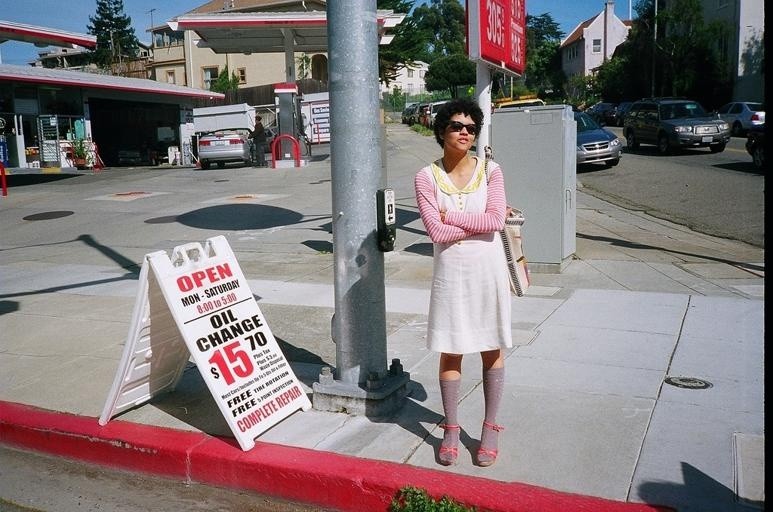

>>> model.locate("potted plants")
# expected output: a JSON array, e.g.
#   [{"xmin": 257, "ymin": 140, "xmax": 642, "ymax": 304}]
[{"xmin": 74, "ymin": 137, "xmax": 86, "ymax": 165}]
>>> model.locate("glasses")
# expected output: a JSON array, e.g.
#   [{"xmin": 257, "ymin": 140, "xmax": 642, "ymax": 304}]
[{"xmin": 449, "ymin": 120, "xmax": 478, "ymax": 135}]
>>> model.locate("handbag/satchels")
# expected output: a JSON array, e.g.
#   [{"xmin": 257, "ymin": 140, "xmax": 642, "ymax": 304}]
[{"xmin": 500, "ymin": 204, "xmax": 530, "ymax": 297}]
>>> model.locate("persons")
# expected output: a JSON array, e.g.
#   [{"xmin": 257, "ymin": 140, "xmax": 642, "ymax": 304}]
[
  {"xmin": 414, "ymin": 99, "xmax": 514, "ymax": 467},
  {"xmin": 249, "ymin": 115, "xmax": 269, "ymax": 167}
]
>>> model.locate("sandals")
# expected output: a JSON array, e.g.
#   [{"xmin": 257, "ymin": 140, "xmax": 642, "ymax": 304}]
[
  {"xmin": 477, "ymin": 422, "xmax": 504, "ymax": 466},
  {"xmin": 439, "ymin": 424, "xmax": 461, "ymax": 466}
]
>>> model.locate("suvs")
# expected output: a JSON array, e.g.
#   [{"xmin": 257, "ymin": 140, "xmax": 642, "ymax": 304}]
[{"xmin": 623, "ymin": 100, "xmax": 731, "ymax": 155}]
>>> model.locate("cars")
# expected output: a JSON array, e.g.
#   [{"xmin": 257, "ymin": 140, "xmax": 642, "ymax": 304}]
[
  {"xmin": 712, "ymin": 101, "xmax": 765, "ymax": 136},
  {"xmin": 746, "ymin": 122, "xmax": 766, "ymax": 172},
  {"xmin": 586, "ymin": 102, "xmax": 633, "ymax": 126},
  {"xmin": 494, "ymin": 98, "xmax": 544, "ymax": 108},
  {"xmin": 401, "ymin": 100, "xmax": 450, "ymax": 130},
  {"xmin": 573, "ymin": 110, "xmax": 622, "ymax": 167},
  {"xmin": 199, "ymin": 130, "xmax": 253, "ymax": 169}
]
